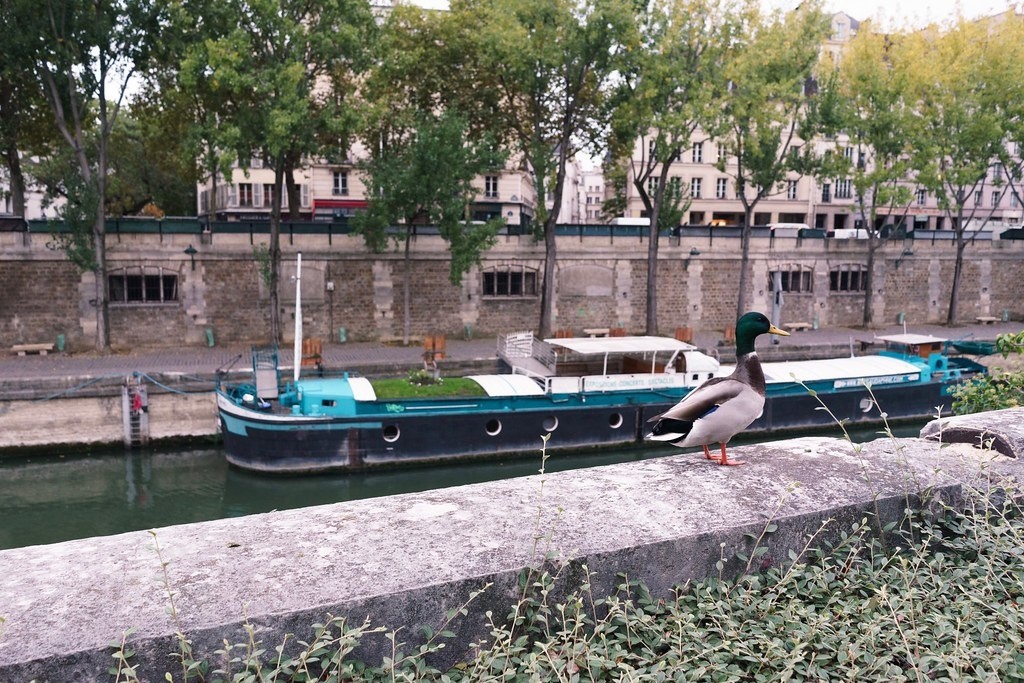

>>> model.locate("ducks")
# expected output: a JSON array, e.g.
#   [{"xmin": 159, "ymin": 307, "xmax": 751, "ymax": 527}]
[{"xmin": 643, "ymin": 311, "xmax": 790, "ymax": 467}]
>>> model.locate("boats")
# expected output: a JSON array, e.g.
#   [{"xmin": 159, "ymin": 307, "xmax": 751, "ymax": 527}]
[
  {"xmin": 212, "ymin": 251, "xmax": 993, "ymax": 474},
  {"xmin": 951, "ymin": 338, "xmax": 1003, "ymax": 355}
]
